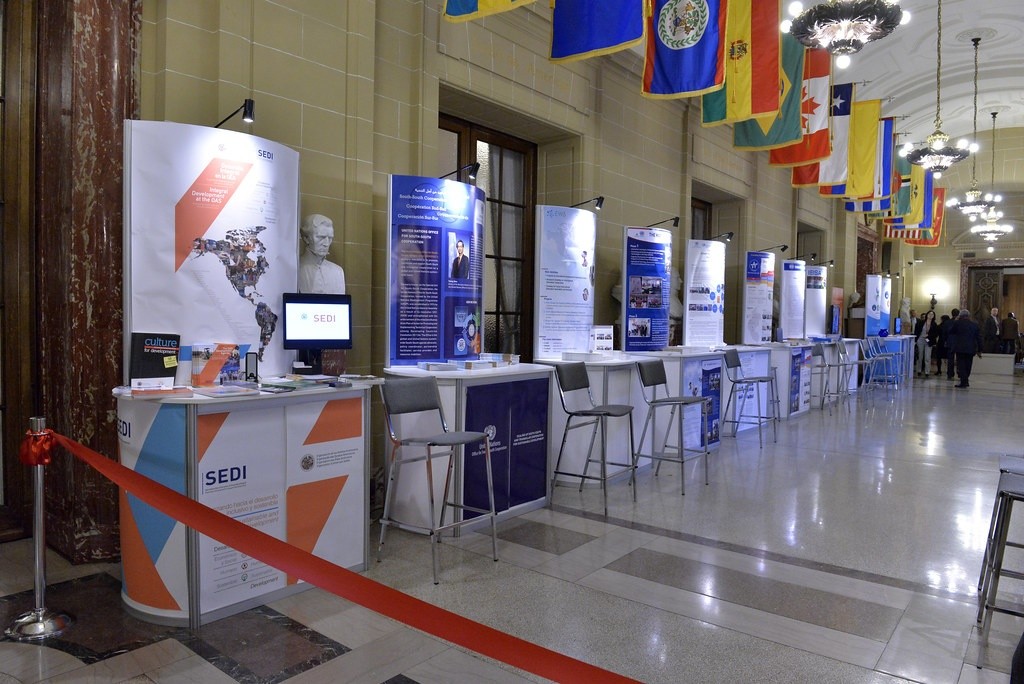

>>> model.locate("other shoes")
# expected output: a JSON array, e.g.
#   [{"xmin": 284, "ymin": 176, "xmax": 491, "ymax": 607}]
[
  {"xmin": 934, "ymin": 370, "xmax": 942, "ymax": 375},
  {"xmin": 955, "ymin": 377, "xmax": 968, "ymax": 388},
  {"xmin": 918, "ymin": 372, "xmax": 922, "ymax": 376},
  {"xmin": 925, "ymin": 373, "xmax": 929, "ymax": 377}
]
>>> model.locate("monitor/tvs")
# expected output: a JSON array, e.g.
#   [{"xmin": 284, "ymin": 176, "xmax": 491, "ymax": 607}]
[
  {"xmin": 830, "ymin": 305, "xmax": 840, "ymax": 334},
  {"xmin": 282, "ymin": 292, "xmax": 352, "ymax": 350},
  {"xmin": 894, "ymin": 317, "xmax": 901, "ymax": 334}
]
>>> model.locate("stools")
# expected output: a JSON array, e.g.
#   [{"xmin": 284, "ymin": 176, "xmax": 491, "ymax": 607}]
[
  {"xmin": 858, "ymin": 339, "xmax": 895, "ymax": 399},
  {"xmin": 811, "ymin": 344, "xmax": 851, "ymax": 415},
  {"xmin": 722, "ymin": 349, "xmax": 777, "ymax": 449},
  {"xmin": 554, "ymin": 363, "xmax": 636, "ymax": 516},
  {"xmin": 377, "ymin": 376, "xmax": 498, "ymax": 585},
  {"xmin": 838, "ymin": 342, "xmax": 872, "ymax": 405},
  {"xmin": 867, "ymin": 337, "xmax": 900, "ymax": 392},
  {"xmin": 875, "ymin": 338, "xmax": 906, "ymax": 386},
  {"xmin": 637, "ymin": 360, "xmax": 708, "ymax": 495},
  {"xmin": 977, "ymin": 455, "xmax": 1024, "ymax": 666}
]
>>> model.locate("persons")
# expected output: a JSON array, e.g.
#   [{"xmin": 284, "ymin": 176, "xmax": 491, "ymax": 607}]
[
  {"xmin": 985, "ymin": 307, "xmax": 1021, "ymax": 354},
  {"xmin": 909, "ymin": 308, "xmax": 960, "ymax": 381},
  {"xmin": 629, "ymin": 324, "xmax": 647, "ymax": 336},
  {"xmin": 949, "ymin": 309, "xmax": 981, "ymax": 387},
  {"xmin": 451, "ymin": 240, "xmax": 469, "ymax": 279},
  {"xmin": 298, "ymin": 214, "xmax": 345, "ymax": 294},
  {"xmin": 899, "ymin": 297, "xmax": 911, "ymax": 322}
]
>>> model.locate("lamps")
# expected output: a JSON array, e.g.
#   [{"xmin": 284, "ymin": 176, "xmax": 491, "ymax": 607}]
[
  {"xmin": 440, "ymin": 163, "xmax": 478, "ymax": 181},
  {"xmin": 214, "ymin": 98, "xmax": 254, "ymax": 128},
  {"xmin": 648, "ymin": 216, "xmax": 835, "ymax": 267},
  {"xmin": 570, "ymin": 196, "xmax": 605, "ymax": 209},
  {"xmin": 779, "ymin": 0, "xmax": 979, "ymax": 179},
  {"xmin": 972, "ymin": 112, "xmax": 1012, "ymax": 253},
  {"xmin": 879, "ymin": 270, "xmax": 900, "ymax": 278},
  {"xmin": 947, "ymin": 37, "xmax": 1002, "ymax": 223}
]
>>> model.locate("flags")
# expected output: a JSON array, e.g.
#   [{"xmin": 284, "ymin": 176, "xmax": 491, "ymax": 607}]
[
  {"xmin": 641, "ymin": 0, "xmax": 730, "ymax": 100},
  {"xmin": 548, "ymin": 0, "xmax": 647, "ymax": 64},
  {"xmin": 442, "ymin": 0, "xmax": 537, "ymax": 24},
  {"xmin": 700, "ymin": 0, "xmax": 946, "ymax": 246}
]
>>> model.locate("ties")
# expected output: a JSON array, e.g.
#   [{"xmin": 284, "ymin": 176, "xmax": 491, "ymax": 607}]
[{"xmin": 911, "ymin": 318, "xmax": 915, "ymax": 334}]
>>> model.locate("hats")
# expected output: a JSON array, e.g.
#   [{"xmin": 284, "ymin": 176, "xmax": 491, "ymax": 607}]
[{"xmin": 1008, "ymin": 312, "xmax": 1016, "ymax": 319}]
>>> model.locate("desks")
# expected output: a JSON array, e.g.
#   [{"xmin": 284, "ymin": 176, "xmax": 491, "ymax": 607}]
[
  {"xmin": 113, "ymin": 385, "xmax": 368, "ymax": 627},
  {"xmin": 535, "ymin": 335, "xmax": 915, "ymax": 489},
  {"xmin": 383, "ymin": 363, "xmax": 555, "ymax": 538}
]
